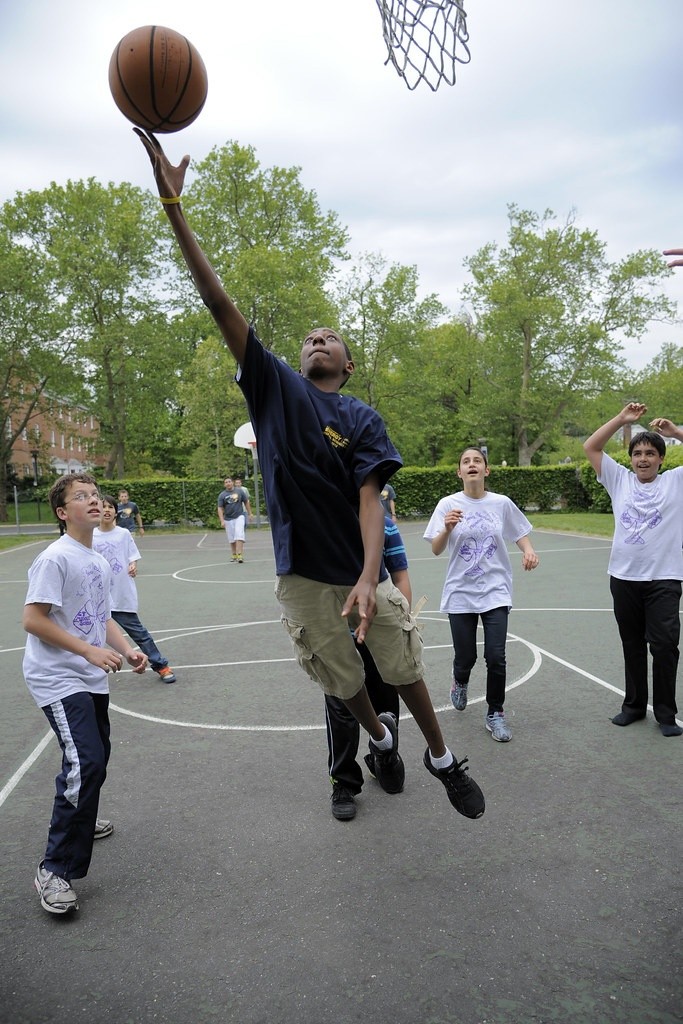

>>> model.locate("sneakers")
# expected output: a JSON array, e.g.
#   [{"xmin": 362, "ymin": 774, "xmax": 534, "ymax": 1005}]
[
  {"xmin": 34, "ymin": 858, "xmax": 79, "ymax": 913},
  {"xmin": 367, "ymin": 713, "xmax": 405, "ymax": 795},
  {"xmin": 423, "ymin": 744, "xmax": 486, "ymax": 819},
  {"xmin": 485, "ymin": 711, "xmax": 512, "ymax": 742},
  {"xmin": 450, "ymin": 668, "xmax": 469, "ymax": 712},
  {"xmin": 328, "ymin": 777, "xmax": 357, "ymax": 819},
  {"xmin": 363, "ymin": 751, "xmax": 376, "ymax": 779},
  {"xmin": 93, "ymin": 818, "xmax": 114, "ymax": 839}
]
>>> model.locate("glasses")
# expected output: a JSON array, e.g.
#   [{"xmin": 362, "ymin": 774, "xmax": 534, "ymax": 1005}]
[{"xmin": 62, "ymin": 492, "xmax": 105, "ymax": 508}]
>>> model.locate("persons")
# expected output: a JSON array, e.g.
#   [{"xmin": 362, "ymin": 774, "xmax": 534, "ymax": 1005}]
[
  {"xmin": 114, "ymin": 490, "xmax": 145, "ymax": 537},
  {"xmin": 133, "ymin": 127, "xmax": 486, "ymax": 819},
  {"xmin": 92, "ymin": 496, "xmax": 176, "ymax": 683},
  {"xmin": 324, "ymin": 517, "xmax": 412, "ymax": 818},
  {"xmin": 380, "ymin": 484, "xmax": 397, "ymax": 524},
  {"xmin": 583, "ymin": 402, "xmax": 683, "ymax": 737},
  {"xmin": 22, "ymin": 473, "xmax": 148, "ymax": 912},
  {"xmin": 422, "ymin": 447, "xmax": 540, "ymax": 742},
  {"xmin": 218, "ymin": 477, "xmax": 253, "ymax": 562}
]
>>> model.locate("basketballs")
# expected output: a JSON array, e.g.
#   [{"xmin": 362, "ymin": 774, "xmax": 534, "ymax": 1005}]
[{"xmin": 109, "ymin": 24, "xmax": 210, "ymax": 136}]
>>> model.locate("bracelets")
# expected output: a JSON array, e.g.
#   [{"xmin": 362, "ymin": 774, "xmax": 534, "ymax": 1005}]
[
  {"xmin": 392, "ymin": 514, "xmax": 396, "ymax": 516},
  {"xmin": 139, "ymin": 527, "xmax": 143, "ymax": 528},
  {"xmin": 159, "ymin": 196, "xmax": 181, "ymax": 204}
]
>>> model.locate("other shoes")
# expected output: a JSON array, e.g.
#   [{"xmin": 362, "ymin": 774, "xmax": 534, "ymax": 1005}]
[
  {"xmin": 229, "ymin": 553, "xmax": 237, "ymax": 562},
  {"xmin": 159, "ymin": 664, "xmax": 176, "ymax": 683},
  {"xmin": 237, "ymin": 552, "xmax": 244, "ymax": 562}
]
